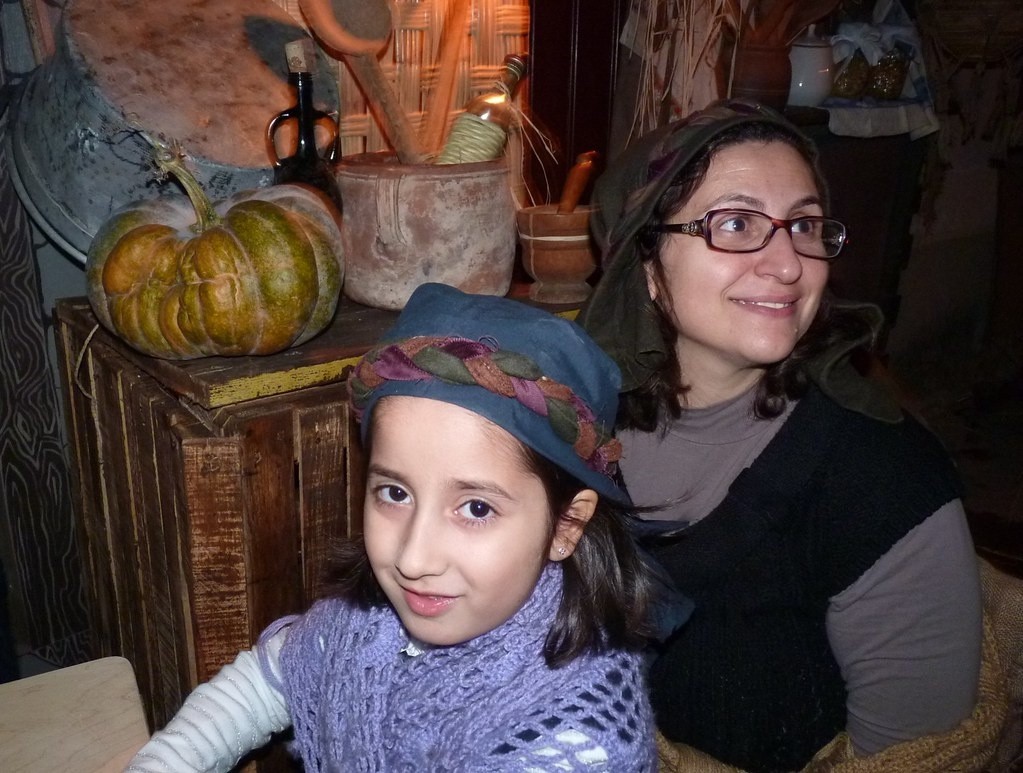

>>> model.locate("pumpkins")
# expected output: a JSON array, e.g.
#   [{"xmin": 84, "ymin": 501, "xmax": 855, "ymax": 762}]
[{"xmin": 86, "ymin": 143, "xmax": 345, "ymax": 362}]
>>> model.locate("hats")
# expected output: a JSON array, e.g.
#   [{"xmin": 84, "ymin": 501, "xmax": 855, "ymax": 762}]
[{"xmin": 343, "ymin": 282, "xmax": 632, "ymax": 506}]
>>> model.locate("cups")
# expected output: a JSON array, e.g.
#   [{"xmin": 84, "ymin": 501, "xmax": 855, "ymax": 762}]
[{"xmin": 337, "ymin": 151, "xmax": 515, "ymax": 309}]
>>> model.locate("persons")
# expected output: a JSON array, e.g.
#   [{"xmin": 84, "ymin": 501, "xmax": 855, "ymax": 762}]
[
  {"xmin": 116, "ymin": 282, "xmax": 652, "ymax": 772},
  {"xmin": 568, "ymin": 101, "xmax": 1011, "ymax": 773}
]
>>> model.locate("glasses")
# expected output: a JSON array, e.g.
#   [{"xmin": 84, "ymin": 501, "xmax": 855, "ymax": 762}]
[{"xmin": 639, "ymin": 208, "xmax": 853, "ymax": 260}]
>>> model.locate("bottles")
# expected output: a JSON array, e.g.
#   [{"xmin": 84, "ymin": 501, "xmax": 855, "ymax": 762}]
[
  {"xmin": 832, "ymin": 47, "xmax": 871, "ymax": 97},
  {"xmin": 870, "ymin": 39, "xmax": 916, "ymax": 102},
  {"xmin": 266, "ymin": 73, "xmax": 344, "ymax": 234},
  {"xmin": 433, "ymin": 51, "xmax": 530, "ymax": 164}
]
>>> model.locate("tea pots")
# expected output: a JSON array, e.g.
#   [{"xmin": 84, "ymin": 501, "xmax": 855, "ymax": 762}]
[{"xmin": 787, "ymin": 24, "xmax": 855, "ymax": 109}]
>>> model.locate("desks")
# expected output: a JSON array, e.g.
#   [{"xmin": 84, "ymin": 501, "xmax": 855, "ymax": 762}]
[{"xmin": 778, "ymin": 105, "xmax": 942, "ymax": 327}]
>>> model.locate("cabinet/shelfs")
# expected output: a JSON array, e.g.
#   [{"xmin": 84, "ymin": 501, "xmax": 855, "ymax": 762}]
[{"xmin": 55, "ymin": 288, "xmax": 583, "ymax": 773}]
